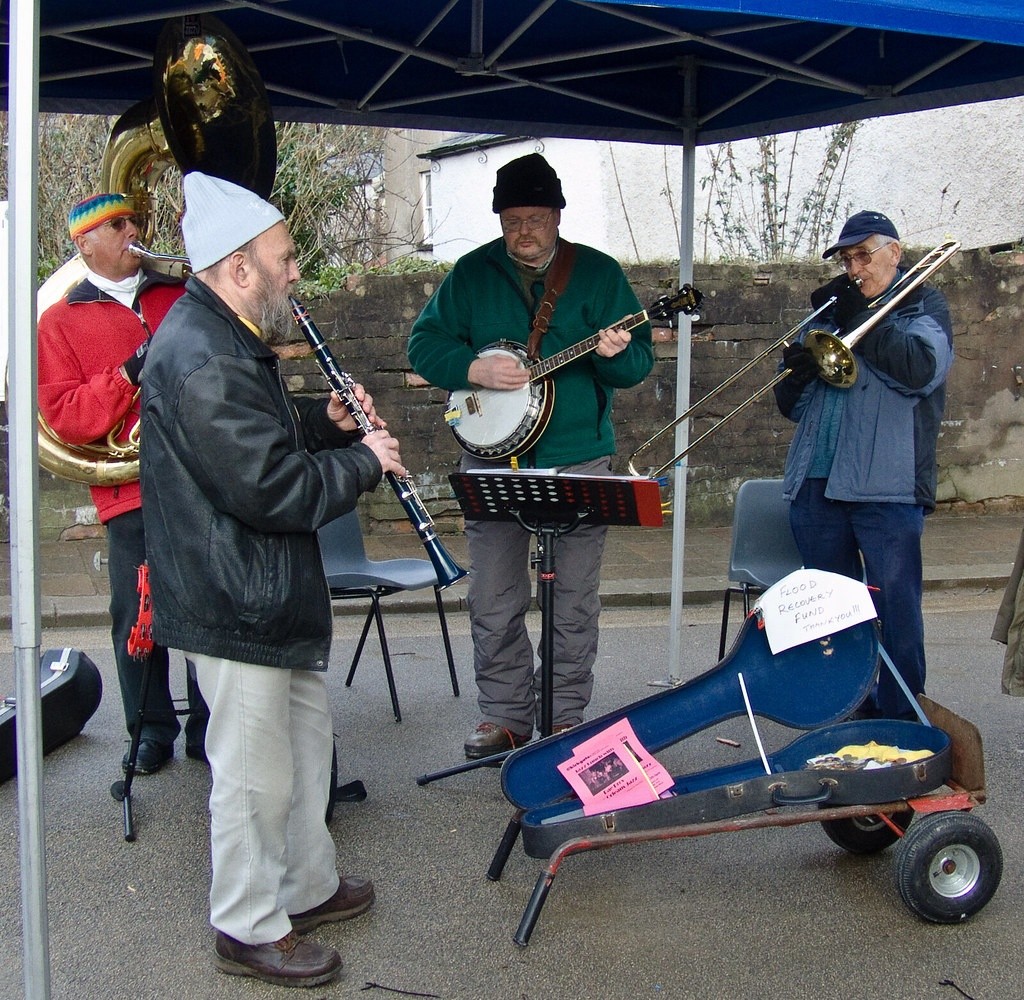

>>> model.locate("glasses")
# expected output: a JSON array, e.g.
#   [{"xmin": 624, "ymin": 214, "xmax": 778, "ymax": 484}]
[
  {"xmin": 80, "ymin": 215, "xmax": 140, "ymax": 235},
  {"xmin": 499, "ymin": 208, "xmax": 554, "ymax": 232},
  {"xmin": 836, "ymin": 241, "xmax": 892, "ymax": 272}
]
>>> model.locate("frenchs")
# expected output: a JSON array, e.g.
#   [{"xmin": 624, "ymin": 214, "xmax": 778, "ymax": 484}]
[{"xmin": 38, "ymin": 11, "xmax": 282, "ymax": 489}]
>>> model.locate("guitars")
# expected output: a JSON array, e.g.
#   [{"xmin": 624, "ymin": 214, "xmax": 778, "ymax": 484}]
[{"xmin": 442, "ymin": 282, "xmax": 706, "ymax": 462}]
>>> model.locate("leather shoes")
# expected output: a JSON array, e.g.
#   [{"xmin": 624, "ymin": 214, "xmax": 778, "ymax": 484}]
[
  {"xmin": 282, "ymin": 875, "xmax": 376, "ymax": 936},
  {"xmin": 463, "ymin": 721, "xmax": 532, "ymax": 760},
  {"xmin": 214, "ymin": 929, "xmax": 343, "ymax": 987}
]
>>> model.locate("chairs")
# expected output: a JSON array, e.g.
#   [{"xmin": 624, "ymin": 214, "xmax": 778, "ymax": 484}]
[
  {"xmin": 318, "ymin": 508, "xmax": 461, "ymax": 723},
  {"xmin": 719, "ymin": 479, "xmax": 804, "ymax": 666}
]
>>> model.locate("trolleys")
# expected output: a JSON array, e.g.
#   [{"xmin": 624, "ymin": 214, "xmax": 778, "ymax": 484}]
[{"xmin": 485, "ymin": 694, "xmax": 1005, "ymax": 948}]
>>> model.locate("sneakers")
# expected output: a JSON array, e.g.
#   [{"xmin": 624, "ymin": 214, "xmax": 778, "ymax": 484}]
[{"xmin": 122, "ymin": 741, "xmax": 174, "ymax": 774}]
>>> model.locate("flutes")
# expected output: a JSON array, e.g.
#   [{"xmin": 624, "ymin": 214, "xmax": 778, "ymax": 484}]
[{"xmin": 288, "ymin": 295, "xmax": 470, "ymax": 594}]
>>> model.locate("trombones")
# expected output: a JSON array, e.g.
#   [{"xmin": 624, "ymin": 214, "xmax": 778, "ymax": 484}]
[{"xmin": 625, "ymin": 238, "xmax": 964, "ymax": 483}]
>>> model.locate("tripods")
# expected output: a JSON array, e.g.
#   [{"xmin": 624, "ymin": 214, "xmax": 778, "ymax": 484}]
[{"xmin": 416, "ymin": 471, "xmax": 663, "ymax": 881}]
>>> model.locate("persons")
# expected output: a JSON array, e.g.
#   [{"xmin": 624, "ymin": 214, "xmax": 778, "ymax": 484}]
[
  {"xmin": 773, "ymin": 211, "xmax": 953, "ymax": 720},
  {"xmin": 143, "ymin": 169, "xmax": 405, "ymax": 985},
  {"xmin": 403, "ymin": 152, "xmax": 654, "ymax": 759},
  {"xmin": 34, "ymin": 195, "xmax": 211, "ymax": 775}
]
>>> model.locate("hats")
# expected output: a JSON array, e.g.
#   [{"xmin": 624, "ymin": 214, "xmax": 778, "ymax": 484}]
[
  {"xmin": 180, "ymin": 171, "xmax": 286, "ymax": 275},
  {"xmin": 68, "ymin": 192, "xmax": 136, "ymax": 242},
  {"xmin": 492, "ymin": 153, "xmax": 566, "ymax": 214},
  {"xmin": 822, "ymin": 209, "xmax": 900, "ymax": 259}
]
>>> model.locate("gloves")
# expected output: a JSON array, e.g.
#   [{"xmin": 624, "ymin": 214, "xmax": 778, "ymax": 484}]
[
  {"xmin": 121, "ymin": 331, "xmax": 154, "ymax": 387},
  {"xmin": 831, "ymin": 281, "xmax": 869, "ymax": 330},
  {"xmin": 782, "ymin": 341, "xmax": 815, "ymax": 369}
]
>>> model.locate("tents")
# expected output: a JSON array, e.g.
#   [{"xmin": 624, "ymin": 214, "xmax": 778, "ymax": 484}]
[{"xmin": 1, "ymin": 1, "xmax": 1024, "ymax": 997}]
